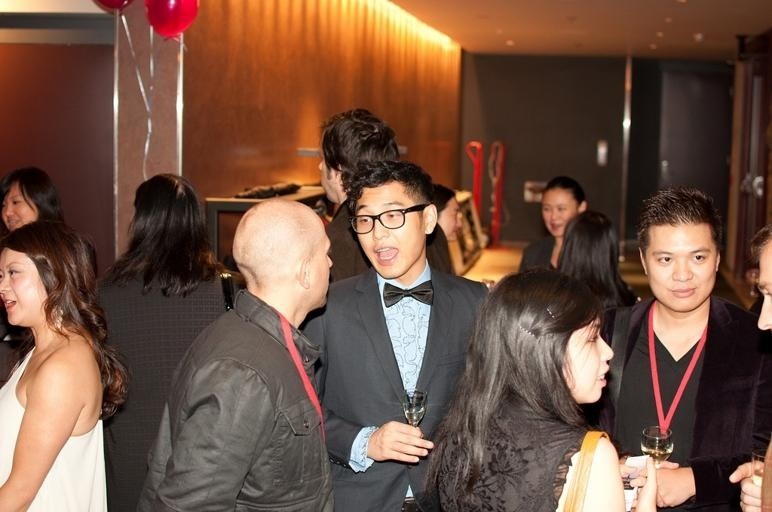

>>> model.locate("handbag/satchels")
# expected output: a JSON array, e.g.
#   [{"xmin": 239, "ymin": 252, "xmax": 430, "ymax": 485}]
[
  {"xmin": 219, "ymin": 271, "xmax": 235, "ymax": 310},
  {"xmin": 561, "ymin": 427, "xmax": 612, "ymax": 512}
]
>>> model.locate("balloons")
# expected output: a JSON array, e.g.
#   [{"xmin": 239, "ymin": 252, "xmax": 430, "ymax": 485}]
[
  {"xmin": 93, "ymin": 0, "xmax": 136, "ymax": 12},
  {"xmin": 143, "ymin": 1, "xmax": 201, "ymax": 41}
]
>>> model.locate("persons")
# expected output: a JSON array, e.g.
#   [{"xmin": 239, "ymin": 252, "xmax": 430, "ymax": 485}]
[
  {"xmin": 1, "ymin": 163, "xmax": 99, "ymax": 386},
  {"xmin": 431, "ymin": 182, "xmax": 462, "ymax": 246},
  {"xmin": 515, "ymin": 171, "xmax": 584, "ymax": 271},
  {"xmin": 419, "ymin": 265, "xmax": 658, "ymax": 512},
  {"xmin": 134, "ymin": 194, "xmax": 335, "ymax": 512},
  {"xmin": 90, "ymin": 174, "xmax": 247, "ymax": 512},
  {"xmin": 597, "ymin": 182, "xmax": 772, "ymax": 512},
  {"xmin": 298, "ymin": 157, "xmax": 492, "ymax": 512},
  {"xmin": 728, "ymin": 221, "xmax": 772, "ymax": 510},
  {"xmin": 554, "ymin": 207, "xmax": 641, "ymax": 421},
  {"xmin": 0, "ymin": 216, "xmax": 133, "ymax": 512},
  {"xmin": 313, "ymin": 103, "xmax": 454, "ymax": 283}
]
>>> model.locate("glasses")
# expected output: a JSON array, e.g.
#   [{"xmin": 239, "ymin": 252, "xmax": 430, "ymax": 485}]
[{"xmin": 348, "ymin": 203, "xmax": 432, "ymax": 234}]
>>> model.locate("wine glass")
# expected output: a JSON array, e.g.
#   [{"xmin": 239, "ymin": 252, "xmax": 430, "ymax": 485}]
[
  {"xmin": 639, "ymin": 424, "xmax": 677, "ymax": 467},
  {"xmin": 404, "ymin": 390, "xmax": 429, "ymax": 425}
]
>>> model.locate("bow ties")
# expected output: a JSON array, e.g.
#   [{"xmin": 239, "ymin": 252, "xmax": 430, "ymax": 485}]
[{"xmin": 382, "ymin": 280, "xmax": 434, "ymax": 308}]
